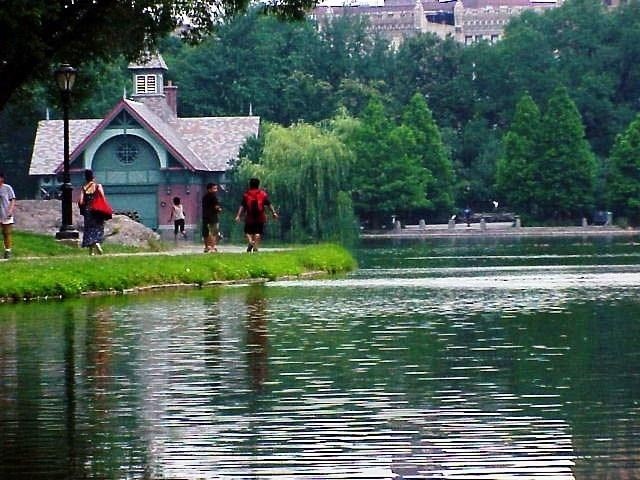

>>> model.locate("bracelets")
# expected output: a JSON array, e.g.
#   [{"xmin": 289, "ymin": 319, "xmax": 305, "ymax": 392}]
[{"xmin": 272, "ymin": 212, "xmax": 277, "ymax": 214}]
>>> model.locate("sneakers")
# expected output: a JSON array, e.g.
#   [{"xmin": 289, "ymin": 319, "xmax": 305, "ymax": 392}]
[
  {"xmin": 89, "ymin": 251, "xmax": 95, "ymax": 256},
  {"xmin": 247, "ymin": 242, "xmax": 253, "ymax": 252},
  {"xmin": 4, "ymin": 251, "xmax": 12, "ymax": 259},
  {"xmin": 95, "ymin": 243, "xmax": 103, "ymax": 255}
]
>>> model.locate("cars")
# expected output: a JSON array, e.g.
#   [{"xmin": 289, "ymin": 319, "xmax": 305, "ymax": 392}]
[
  {"xmin": 458, "ymin": 210, "xmax": 516, "ymax": 222},
  {"xmin": 551, "ymin": 204, "xmax": 607, "ymax": 226}
]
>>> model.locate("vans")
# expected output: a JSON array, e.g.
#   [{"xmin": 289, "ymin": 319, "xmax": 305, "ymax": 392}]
[{"xmin": 352, "ymin": 211, "xmax": 400, "ymax": 231}]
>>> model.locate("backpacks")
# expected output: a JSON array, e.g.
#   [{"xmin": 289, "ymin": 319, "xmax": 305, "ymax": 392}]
[{"xmin": 245, "ymin": 190, "xmax": 266, "ymax": 224}]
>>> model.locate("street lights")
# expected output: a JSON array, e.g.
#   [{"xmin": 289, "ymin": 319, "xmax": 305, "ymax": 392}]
[{"xmin": 53, "ymin": 59, "xmax": 79, "ymax": 239}]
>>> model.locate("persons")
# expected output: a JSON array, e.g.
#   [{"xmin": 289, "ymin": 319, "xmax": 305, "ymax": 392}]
[
  {"xmin": 167, "ymin": 196, "xmax": 188, "ymax": 241},
  {"xmin": 202, "ymin": 182, "xmax": 223, "ymax": 253},
  {"xmin": 235, "ymin": 178, "xmax": 279, "ymax": 253},
  {"xmin": 77, "ymin": 168, "xmax": 112, "ymax": 256},
  {"xmin": 0, "ymin": 168, "xmax": 17, "ymax": 260},
  {"xmin": 463, "ymin": 205, "xmax": 474, "ymax": 227}
]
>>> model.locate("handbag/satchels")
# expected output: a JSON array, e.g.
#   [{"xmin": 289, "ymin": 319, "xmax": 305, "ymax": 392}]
[{"xmin": 89, "ymin": 185, "xmax": 113, "ymax": 220}]
